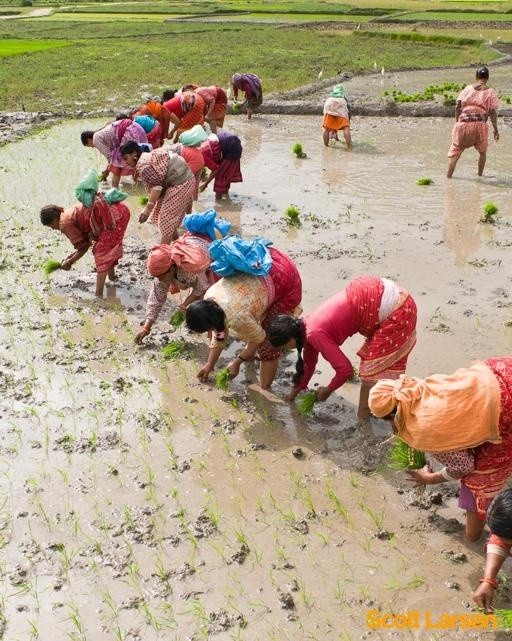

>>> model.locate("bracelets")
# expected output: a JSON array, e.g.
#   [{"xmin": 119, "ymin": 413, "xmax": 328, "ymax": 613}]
[
  {"xmin": 66, "ymin": 260, "xmax": 71, "ymax": 266},
  {"xmin": 237, "ymin": 354, "xmax": 246, "ymax": 362},
  {"xmin": 480, "ymin": 578, "xmax": 498, "ymax": 590},
  {"xmin": 143, "ymin": 328, "xmax": 150, "ymax": 334}
]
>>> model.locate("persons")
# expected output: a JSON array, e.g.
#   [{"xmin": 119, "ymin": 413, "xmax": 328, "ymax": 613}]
[
  {"xmin": 368, "ymin": 356, "xmax": 512, "ymax": 541},
  {"xmin": 134, "ymin": 227, "xmax": 223, "ymax": 346},
  {"xmin": 268, "ymin": 275, "xmax": 417, "ymax": 418},
  {"xmin": 185, "ymin": 247, "xmax": 302, "ymax": 389},
  {"xmin": 446, "ymin": 66, "xmax": 499, "ymax": 177},
  {"xmin": 231, "ymin": 73, "xmax": 262, "ymax": 118},
  {"xmin": 79, "ymin": 83, "xmax": 243, "ymax": 243},
  {"xmin": 473, "ymin": 487, "xmax": 512, "ymax": 614},
  {"xmin": 40, "ymin": 199, "xmax": 130, "ymax": 297},
  {"xmin": 322, "ymin": 82, "xmax": 353, "ymax": 148}
]
